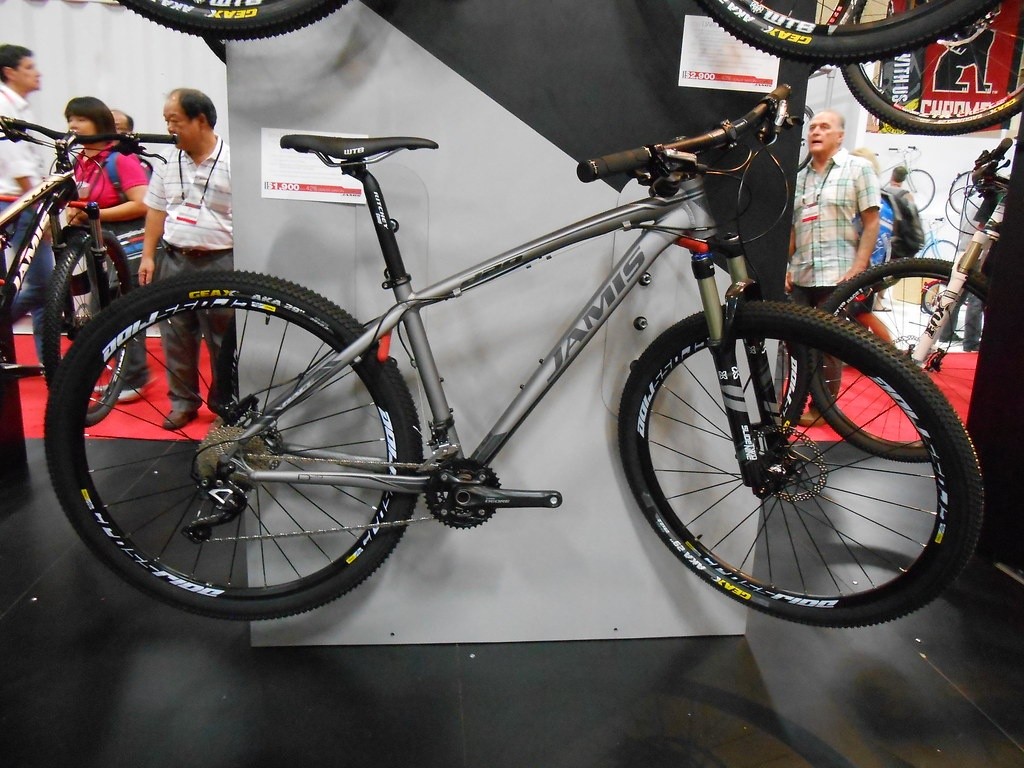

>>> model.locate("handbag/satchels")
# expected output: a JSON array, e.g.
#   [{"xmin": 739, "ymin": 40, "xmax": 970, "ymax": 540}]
[
  {"xmin": 100, "ymin": 151, "xmax": 165, "ymax": 288},
  {"xmin": 849, "ymin": 158, "xmax": 894, "ymax": 270}
]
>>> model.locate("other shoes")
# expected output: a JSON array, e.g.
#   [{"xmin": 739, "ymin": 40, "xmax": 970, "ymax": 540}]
[
  {"xmin": 939, "ymin": 332, "xmax": 964, "ymax": 342},
  {"xmin": 162, "ymin": 409, "xmax": 198, "ymax": 429},
  {"xmin": 797, "ymin": 408, "xmax": 827, "ymax": 426},
  {"xmin": 94, "ymin": 385, "xmax": 109, "ymax": 395},
  {"xmin": 962, "ymin": 341, "xmax": 981, "ymax": 351},
  {"xmin": 116, "ymin": 388, "xmax": 143, "ymax": 402}
]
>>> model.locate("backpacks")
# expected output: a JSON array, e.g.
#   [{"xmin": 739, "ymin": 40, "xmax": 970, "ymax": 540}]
[{"xmin": 881, "ymin": 188, "xmax": 921, "ymax": 258}]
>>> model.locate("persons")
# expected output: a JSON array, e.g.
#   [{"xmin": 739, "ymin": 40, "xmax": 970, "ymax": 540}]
[
  {"xmin": 853, "ymin": 148, "xmax": 992, "ymax": 351},
  {"xmin": 138, "ymin": 89, "xmax": 235, "ymax": 431},
  {"xmin": 784, "ymin": 112, "xmax": 882, "ymax": 427},
  {"xmin": 64, "ymin": 97, "xmax": 154, "ymax": 403},
  {"xmin": 0, "ymin": 44, "xmax": 57, "ymax": 372}
]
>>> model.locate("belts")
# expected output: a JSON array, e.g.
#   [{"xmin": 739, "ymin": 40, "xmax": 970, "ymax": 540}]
[{"xmin": 168, "ymin": 244, "xmax": 222, "ymax": 257}]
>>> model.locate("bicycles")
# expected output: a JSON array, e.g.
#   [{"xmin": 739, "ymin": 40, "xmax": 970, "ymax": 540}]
[
  {"xmin": 806, "ymin": 135, "xmax": 1013, "ymax": 465},
  {"xmin": 43, "ymin": 74, "xmax": 988, "ymax": 627},
  {"xmin": 1, "ymin": 116, "xmax": 179, "ymax": 425}
]
